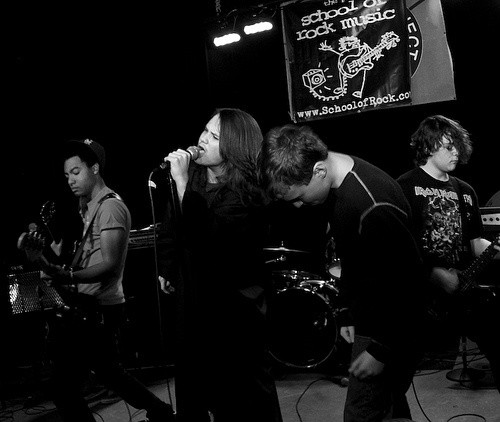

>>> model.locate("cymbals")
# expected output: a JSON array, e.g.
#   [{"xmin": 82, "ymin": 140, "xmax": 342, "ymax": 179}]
[{"xmin": 262, "ymin": 245, "xmax": 305, "ymax": 253}]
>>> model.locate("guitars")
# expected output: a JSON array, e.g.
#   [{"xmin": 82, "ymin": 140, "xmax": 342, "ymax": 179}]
[
  {"xmin": 453, "ymin": 233, "xmax": 500, "ymax": 293},
  {"xmin": 17, "ymin": 232, "xmax": 75, "ymax": 304}
]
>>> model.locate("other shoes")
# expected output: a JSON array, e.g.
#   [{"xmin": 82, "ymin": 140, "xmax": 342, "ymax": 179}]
[{"xmin": 145, "ymin": 404, "xmax": 173, "ymax": 422}]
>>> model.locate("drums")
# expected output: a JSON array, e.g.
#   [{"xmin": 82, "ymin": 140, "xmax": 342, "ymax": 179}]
[
  {"xmin": 272, "ymin": 270, "xmax": 311, "ymax": 290},
  {"xmin": 324, "ymin": 233, "xmax": 342, "ymax": 280},
  {"xmin": 265, "ymin": 286, "xmax": 339, "ymax": 369}
]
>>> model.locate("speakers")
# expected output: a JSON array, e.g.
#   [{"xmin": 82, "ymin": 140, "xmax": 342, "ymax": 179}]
[{"xmin": 111, "ymin": 245, "xmax": 178, "ymax": 373}]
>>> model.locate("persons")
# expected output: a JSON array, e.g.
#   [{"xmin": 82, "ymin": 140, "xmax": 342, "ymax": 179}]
[
  {"xmin": 50, "ymin": 140, "xmax": 174, "ymax": 422},
  {"xmin": 158, "ymin": 108, "xmax": 284, "ymax": 422},
  {"xmin": 393, "ymin": 114, "xmax": 500, "ymax": 393},
  {"xmin": 256, "ymin": 126, "xmax": 445, "ymax": 422}
]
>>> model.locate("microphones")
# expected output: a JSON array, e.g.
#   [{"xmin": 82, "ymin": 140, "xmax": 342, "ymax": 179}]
[{"xmin": 152, "ymin": 146, "xmax": 200, "ymax": 173}]
